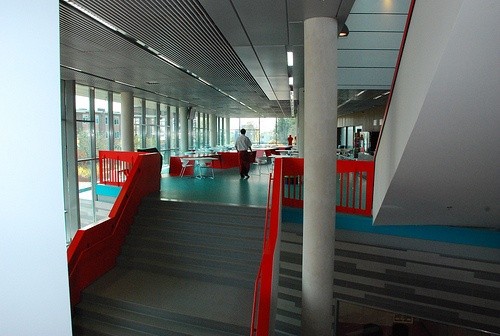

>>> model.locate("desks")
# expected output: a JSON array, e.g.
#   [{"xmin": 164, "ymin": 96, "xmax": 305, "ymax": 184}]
[{"xmin": 171, "ymin": 145, "xmax": 298, "ymax": 180}]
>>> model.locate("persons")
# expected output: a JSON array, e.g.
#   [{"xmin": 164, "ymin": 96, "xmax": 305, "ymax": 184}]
[
  {"xmin": 235, "ymin": 129, "xmax": 252, "ymax": 179},
  {"xmin": 359, "ymin": 134, "xmax": 364, "ymax": 140},
  {"xmin": 287, "ymin": 135, "xmax": 293, "ymax": 146}
]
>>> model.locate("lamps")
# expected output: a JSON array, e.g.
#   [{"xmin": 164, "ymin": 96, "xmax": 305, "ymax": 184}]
[{"xmin": 339, "ymin": 23, "xmax": 350, "ymax": 37}]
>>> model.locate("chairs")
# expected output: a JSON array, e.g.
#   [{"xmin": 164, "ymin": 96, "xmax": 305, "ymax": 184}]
[
  {"xmin": 252, "ymin": 157, "xmax": 269, "ymax": 176},
  {"xmin": 179, "ymin": 159, "xmax": 194, "ymax": 176}
]
